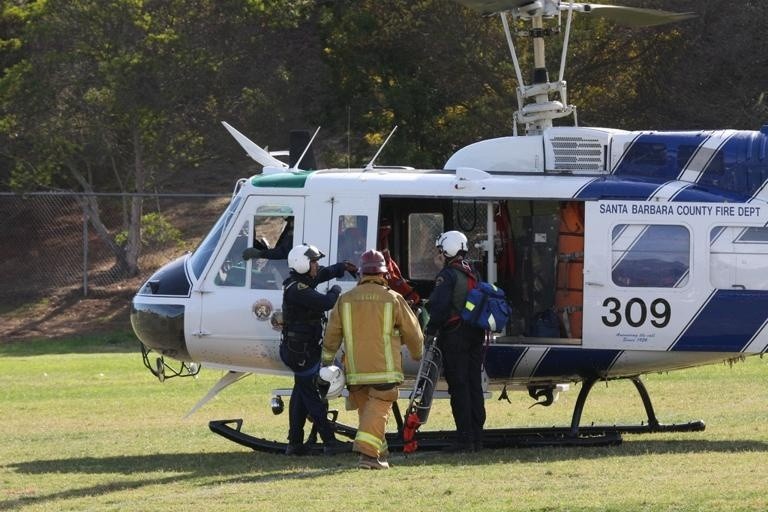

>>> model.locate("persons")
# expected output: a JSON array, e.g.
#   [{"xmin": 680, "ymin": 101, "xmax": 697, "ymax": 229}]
[
  {"xmin": 318, "ymin": 249, "xmax": 424, "ymax": 471},
  {"xmin": 226, "ymin": 207, "xmax": 294, "ymax": 288},
  {"xmin": 424, "ymin": 230, "xmax": 487, "ymax": 454},
  {"xmin": 278, "ymin": 243, "xmax": 360, "ymax": 456}
]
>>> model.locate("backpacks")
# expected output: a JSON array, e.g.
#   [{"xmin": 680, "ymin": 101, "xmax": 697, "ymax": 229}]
[{"xmin": 435, "ymin": 265, "xmax": 515, "ymax": 339}]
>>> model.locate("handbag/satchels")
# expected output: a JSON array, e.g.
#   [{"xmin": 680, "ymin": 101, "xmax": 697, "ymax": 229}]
[{"xmin": 279, "ymin": 338, "xmax": 310, "ymax": 372}]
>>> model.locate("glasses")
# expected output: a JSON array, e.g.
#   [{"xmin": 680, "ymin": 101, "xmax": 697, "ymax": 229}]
[{"xmin": 439, "ymin": 248, "xmax": 443, "ymax": 254}]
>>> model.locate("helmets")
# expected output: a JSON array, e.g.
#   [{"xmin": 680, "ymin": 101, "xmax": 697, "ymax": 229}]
[
  {"xmin": 280, "ymin": 206, "xmax": 293, "ymax": 220},
  {"xmin": 434, "ymin": 230, "xmax": 470, "ymax": 263},
  {"xmin": 287, "ymin": 242, "xmax": 326, "ymax": 278},
  {"xmin": 313, "ymin": 364, "xmax": 346, "ymax": 400}
]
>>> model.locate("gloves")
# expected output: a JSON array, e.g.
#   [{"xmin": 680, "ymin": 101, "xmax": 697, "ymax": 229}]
[
  {"xmin": 342, "ymin": 260, "xmax": 360, "ymax": 278},
  {"xmin": 329, "ymin": 285, "xmax": 342, "ymax": 296},
  {"xmin": 242, "ymin": 247, "xmax": 262, "ymax": 261}
]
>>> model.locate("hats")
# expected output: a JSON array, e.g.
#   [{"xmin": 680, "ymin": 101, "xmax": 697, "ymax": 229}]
[{"xmin": 356, "ymin": 249, "xmax": 388, "ymax": 275}]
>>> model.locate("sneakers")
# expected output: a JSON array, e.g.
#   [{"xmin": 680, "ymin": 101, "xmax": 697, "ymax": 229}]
[
  {"xmin": 285, "ymin": 440, "xmax": 320, "ymax": 458},
  {"xmin": 323, "ymin": 439, "xmax": 352, "ymax": 455},
  {"xmin": 358, "ymin": 453, "xmax": 391, "ymax": 470}
]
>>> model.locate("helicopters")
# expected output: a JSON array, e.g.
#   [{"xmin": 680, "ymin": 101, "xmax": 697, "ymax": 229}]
[{"xmin": 130, "ymin": 0, "xmax": 768, "ymax": 454}]
